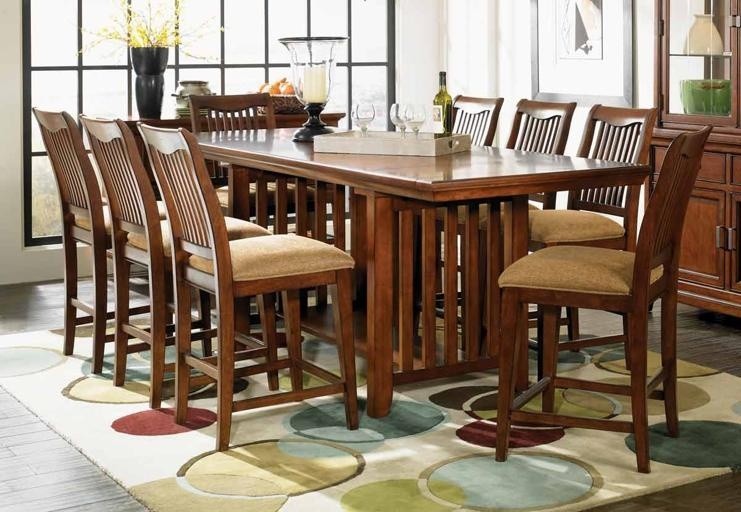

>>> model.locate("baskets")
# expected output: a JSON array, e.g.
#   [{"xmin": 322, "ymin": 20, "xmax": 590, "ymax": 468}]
[{"xmin": 255, "ymin": 92, "xmax": 308, "ymax": 115}]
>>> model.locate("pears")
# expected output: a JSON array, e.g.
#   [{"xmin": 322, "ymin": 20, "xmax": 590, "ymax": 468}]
[{"xmin": 260, "ymin": 77, "xmax": 296, "ymax": 95}]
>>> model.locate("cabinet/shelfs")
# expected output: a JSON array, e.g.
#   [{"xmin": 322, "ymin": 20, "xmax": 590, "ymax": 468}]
[
  {"xmin": 654, "ymin": 0, "xmax": 741, "ymax": 129},
  {"xmin": 642, "ymin": 129, "xmax": 740, "ymax": 327}
]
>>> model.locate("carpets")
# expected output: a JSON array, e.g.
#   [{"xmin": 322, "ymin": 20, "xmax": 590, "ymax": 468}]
[{"xmin": 0, "ymin": 294, "xmax": 741, "ymax": 510}]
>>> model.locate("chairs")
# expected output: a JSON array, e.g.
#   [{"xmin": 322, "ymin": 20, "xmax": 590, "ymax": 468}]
[
  {"xmin": 189, "ymin": 94, "xmax": 330, "ymax": 316},
  {"xmin": 527, "ymin": 103, "xmax": 661, "ymax": 383},
  {"xmin": 74, "ymin": 113, "xmax": 273, "ymax": 409},
  {"xmin": 491, "ymin": 122, "xmax": 712, "ymax": 472},
  {"xmin": 416, "ymin": 99, "xmax": 576, "ymax": 354},
  {"xmin": 135, "ymin": 121, "xmax": 360, "ymax": 450},
  {"xmin": 32, "ymin": 105, "xmax": 173, "ymax": 372},
  {"xmin": 392, "ymin": 96, "xmax": 505, "ymax": 311}
]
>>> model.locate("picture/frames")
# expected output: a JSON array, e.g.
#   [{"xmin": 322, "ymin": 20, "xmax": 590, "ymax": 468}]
[{"xmin": 528, "ymin": 1, "xmax": 636, "ymax": 109}]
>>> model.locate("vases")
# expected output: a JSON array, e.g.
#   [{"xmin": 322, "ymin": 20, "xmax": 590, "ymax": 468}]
[
  {"xmin": 130, "ymin": 47, "xmax": 171, "ymax": 120},
  {"xmin": 684, "ymin": 13, "xmax": 725, "ymax": 55},
  {"xmin": 171, "ymin": 77, "xmax": 216, "ymax": 117}
]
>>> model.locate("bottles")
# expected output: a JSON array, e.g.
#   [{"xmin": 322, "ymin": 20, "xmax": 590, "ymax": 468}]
[{"xmin": 433, "ymin": 72, "xmax": 453, "ymax": 148}]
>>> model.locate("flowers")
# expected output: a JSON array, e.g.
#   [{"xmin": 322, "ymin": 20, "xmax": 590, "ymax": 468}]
[{"xmin": 77, "ymin": 0, "xmax": 226, "ymax": 65}]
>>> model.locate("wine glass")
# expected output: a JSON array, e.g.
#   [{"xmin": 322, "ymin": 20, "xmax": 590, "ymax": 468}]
[
  {"xmin": 403, "ymin": 103, "xmax": 427, "ymax": 142},
  {"xmin": 356, "ymin": 103, "xmax": 376, "ymax": 136},
  {"xmin": 352, "ymin": 111, "xmax": 367, "ymax": 140},
  {"xmin": 391, "ymin": 104, "xmax": 409, "ymax": 142}
]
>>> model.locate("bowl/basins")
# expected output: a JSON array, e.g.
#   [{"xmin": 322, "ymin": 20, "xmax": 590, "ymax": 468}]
[{"xmin": 248, "ymin": 94, "xmax": 329, "ymax": 115}]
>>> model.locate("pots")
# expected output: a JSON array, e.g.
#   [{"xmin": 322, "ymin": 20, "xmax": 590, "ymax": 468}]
[{"xmin": 175, "ymin": 81, "xmax": 214, "ymax": 117}]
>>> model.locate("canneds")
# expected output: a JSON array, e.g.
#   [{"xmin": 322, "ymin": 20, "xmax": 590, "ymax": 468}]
[{"xmin": 176, "ymin": 81, "xmax": 211, "ymax": 108}]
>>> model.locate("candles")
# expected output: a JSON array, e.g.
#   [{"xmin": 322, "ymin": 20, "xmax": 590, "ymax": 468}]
[{"xmin": 303, "ymin": 67, "xmax": 326, "ymax": 102}]
